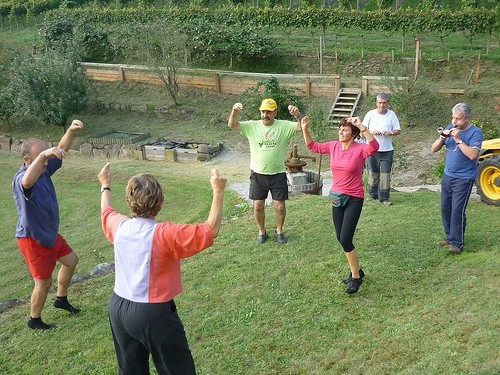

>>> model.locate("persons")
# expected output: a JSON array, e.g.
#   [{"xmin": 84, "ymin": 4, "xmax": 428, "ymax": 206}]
[
  {"xmin": 227, "ymin": 99, "xmax": 303, "ymax": 245},
  {"xmin": 300, "ymin": 116, "xmax": 381, "ymax": 294},
  {"xmin": 430, "ymin": 103, "xmax": 484, "ymax": 254},
  {"xmin": 96, "ymin": 163, "xmax": 227, "ymax": 375},
  {"xmin": 361, "ymin": 93, "xmax": 401, "ymax": 206},
  {"xmin": 10, "ymin": 119, "xmax": 84, "ymax": 330}
]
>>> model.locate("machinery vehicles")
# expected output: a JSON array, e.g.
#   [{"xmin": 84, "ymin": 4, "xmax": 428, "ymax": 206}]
[{"xmin": 475, "ymin": 130, "xmax": 500, "ymax": 206}]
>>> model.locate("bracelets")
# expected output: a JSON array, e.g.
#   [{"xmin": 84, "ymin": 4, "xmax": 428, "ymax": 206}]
[
  {"xmin": 294, "ymin": 112, "xmax": 301, "ymax": 120},
  {"xmin": 360, "ymin": 125, "xmax": 367, "ymax": 132},
  {"xmin": 390, "ymin": 130, "xmax": 395, "ymax": 136}
]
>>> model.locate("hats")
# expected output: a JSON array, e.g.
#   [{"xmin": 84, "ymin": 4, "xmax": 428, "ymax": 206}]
[{"xmin": 258, "ymin": 98, "xmax": 278, "ymax": 111}]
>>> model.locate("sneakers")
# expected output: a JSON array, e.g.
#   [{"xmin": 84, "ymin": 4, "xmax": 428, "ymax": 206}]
[
  {"xmin": 366, "ymin": 196, "xmax": 374, "ymax": 200},
  {"xmin": 345, "ymin": 274, "xmax": 362, "ymax": 293},
  {"xmin": 448, "ymin": 245, "xmax": 461, "ymax": 254},
  {"xmin": 256, "ymin": 234, "xmax": 268, "ymax": 243},
  {"xmin": 274, "ymin": 229, "xmax": 287, "ymax": 243},
  {"xmin": 383, "ymin": 200, "xmax": 392, "ymax": 205},
  {"xmin": 438, "ymin": 240, "xmax": 450, "ymax": 246},
  {"xmin": 342, "ymin": 269, "xmax": 364, "ymax": 284}
]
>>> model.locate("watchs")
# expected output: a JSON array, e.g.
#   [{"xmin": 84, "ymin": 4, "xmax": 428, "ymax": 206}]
[
  {"xmin": 100, "ymin": 187, "xmax": 111, "ymax": 193},
  {"xmin": 456, "ymin": 140, "xmax": 464, "ymax": 146}
]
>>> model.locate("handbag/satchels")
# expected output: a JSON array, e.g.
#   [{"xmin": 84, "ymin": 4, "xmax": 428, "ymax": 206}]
[{"xmin": 328, "ymin": 190, "xmax": 350, "ymax": 207}]
